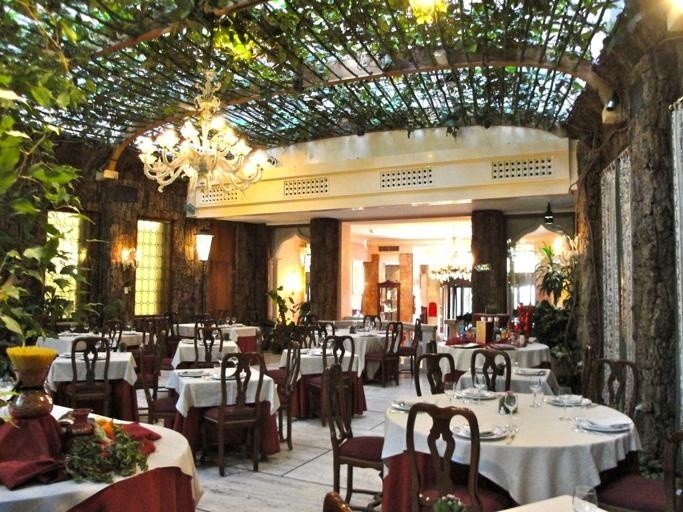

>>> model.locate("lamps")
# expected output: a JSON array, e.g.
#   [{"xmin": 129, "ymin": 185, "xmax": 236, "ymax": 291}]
[
  {"xmin": 196, "ymin": 230, "xmax": 214, "ymax": 321},
  {"xmin": 544, "ymin": 202, "xmax": 554, "ymax": 225},
  {"xmin": 135, "ymin": 13, "xmax": 268, "ymax": 196},
  {"xmin": 122, "ymin": 247, "xmax": 143, "ymax": 273}
]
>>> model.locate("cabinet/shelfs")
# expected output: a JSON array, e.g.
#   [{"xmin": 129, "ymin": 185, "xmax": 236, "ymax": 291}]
[{"xmin": 377, "ymin": 280, "xmax": 400, "ymax": 321}]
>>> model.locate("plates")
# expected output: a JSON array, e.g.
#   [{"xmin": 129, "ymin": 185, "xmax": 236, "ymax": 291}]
[
  {"xmin": 393, "ymin": 403, "xmax": 410, "ymax": 410},
  {"xmin": 59, "ymin": 350, "xmax": 107, "ymax": 361},
  {"xmin": 179, "ymin": 372, "xmax": 246, "ymax": 377},
  {"xmin": 457, "ymin": 391, "xmax": 496, "ymax": 399},
  {"xmin": 581, "ymin": 418, "xmax": 629, "ymax": 432},
  {"xmin": 452, "ymin": 425, "xmax": 508, "ymax": 439}
]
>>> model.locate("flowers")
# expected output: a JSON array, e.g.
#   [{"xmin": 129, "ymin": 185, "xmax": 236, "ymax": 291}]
[{"xmin": 513, "ymin": 302, "xmax": 535, "ymax": 343}]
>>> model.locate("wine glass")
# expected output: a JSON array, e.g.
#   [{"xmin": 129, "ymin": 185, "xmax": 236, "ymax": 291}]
[
  {"xmin": 573, "ymin": 485, "xmax": 598, "ymax": 512},
  {"xmin": 443, "ymin": 377, "xmax": 572, "ymax": 429},
  {"xmin": 318, "ymin": 337, "xmax": 324, "ymax": 349},
  {"xmin": 69, "ymin": 322, "xmax": 91, "ymax": 338},
  {"xmin": 212, "ymin": 330, "xmax": 218, "ymax": 338},
  {"xmin": 370, "ymin": 321, "xmax": 374, "ymax": 329},
  {"xmin": 225, "ymin": 316, "xmax": 238, "ymax": 323},
  {"xmin": 217, "ymin": 353, "xmax": 223, "ymax": 372}
]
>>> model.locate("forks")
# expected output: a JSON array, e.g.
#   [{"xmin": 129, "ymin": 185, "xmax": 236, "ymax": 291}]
[{"xmin": 546, "ymin": 398, "xmax": 592, "ymax": 406}]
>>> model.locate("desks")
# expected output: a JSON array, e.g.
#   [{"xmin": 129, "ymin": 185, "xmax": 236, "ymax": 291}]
[
  {"xmin": 45, "ymin": 351, "xmax": 139, "ymax": 422},
  {"xmin": 37, "ymin": 333, "xmax": 117, "ymax": 355},
  {"xmin": 0, "ymin": 389, "xmax": 204, "ymax": 512}
]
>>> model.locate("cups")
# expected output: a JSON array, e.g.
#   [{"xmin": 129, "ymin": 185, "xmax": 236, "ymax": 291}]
[
  {"xmin": 461, "ymin": 389, "xmax": 473, "ymax": 403},
  {"xmin": 475, "ymin": 320, "xmax": 487, "ymax": 343},
  {"xmin": 574, "ymin": 416, "xmax": 586, "ymax": 433}
]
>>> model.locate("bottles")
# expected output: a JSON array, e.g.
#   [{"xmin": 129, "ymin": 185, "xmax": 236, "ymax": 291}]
[{"xmin": 482, "ymin": 317, "xmax": 502, "ymax": 345}]
